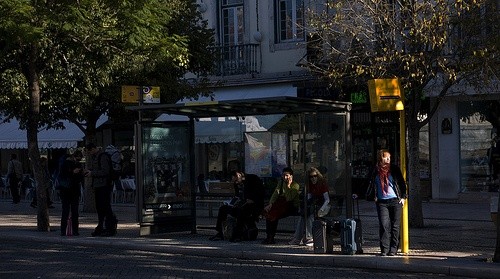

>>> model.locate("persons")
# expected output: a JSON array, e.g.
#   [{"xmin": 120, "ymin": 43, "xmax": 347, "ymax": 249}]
[
  {"xmin": 22, "ymin": 157, "xmax": 56, "ymax": 208},
  {"xmin": 60, "ymin": 150, "xmax": 85, "ymax": 236},
  {"xmin": 5, "ymin": 153, "xmax": 23, "ymax": 204},
  {"xmin": 285, "ymin": 167, "xmax": 330, "ymax": 246},
  {"xmin": 372, "ymin": 149, "xmax": 407, "ymax": 256},
  {"xmin": 210, "ymin": 166, "xmax": 218, "ymax": 179},
  {"xmin": 486, "ymin": 141, "xmax": 496, "ymax": 182},
  {"xmin": 256, "ymin": 167, "xmax": 299, "ymax": 244},
  {"xmin": 84, "ymin": 142, "xmax": 116, "ymax": 236},
  {"xmin": 209, "ymin": 169, "xmax": 265, "ymax": 242}
]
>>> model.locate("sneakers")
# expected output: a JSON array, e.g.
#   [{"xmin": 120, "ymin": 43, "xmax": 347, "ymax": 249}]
[
  {"xmin": 299, "ymin": 238, "xmax": 305, "ymax": 245},
  {"xmin": 288, "ymin": 238, "xmax": 300, "ymax": 244}
]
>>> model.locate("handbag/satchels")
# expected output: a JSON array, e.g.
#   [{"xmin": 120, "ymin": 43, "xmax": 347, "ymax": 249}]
[
  {"xmin": 8, "ymin": 160, "xmax": 18, "ymax": 184},
  {"xmin": 270, "ymin": 195, "xmax": 287, "ymax": 221},
  {"xmin": 66, "ymin": 217, "xmax": 73, "ymax": 236},
  {"xmin": 365, "ymin": 182, "xmax": 374, "ymax": 201}
]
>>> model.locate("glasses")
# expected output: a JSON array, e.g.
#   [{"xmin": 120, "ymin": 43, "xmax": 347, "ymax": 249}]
[{"xmin": 308, "ymin": 175, "xmax": 318, "ymax": 178}]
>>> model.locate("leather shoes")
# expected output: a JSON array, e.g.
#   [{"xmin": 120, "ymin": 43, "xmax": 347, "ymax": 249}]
[
  {"xmin": 209, "ymin": 234, "xmax": 224, "ymax": 241},
  {"xmin": 229, "ymin": 237, "xmax": 242, "ymax": 242}
]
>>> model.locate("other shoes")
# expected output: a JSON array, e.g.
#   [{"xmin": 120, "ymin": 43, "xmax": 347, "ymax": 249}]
[
  {"xmin": 261, "ymin": 238, "xmax": 275, "ymax": 245},
  {"xmin": 48, "ymin": 205, "xmax": 55, "ymax": 208},
  {"xmin": 389, "ymin": 253, "xmax": 395, "ymax": 256},
  {"xmin": 74, "ymin": 232, "xmax": 80, "ymax": 236},
  {"xmin": 30, "ymin": 203, "xmax": 37, "ymax": 208},
  {"xmin": 91, "ymin": 229, "xmax": 102, "ymax": 236},
  {"xmin": 381, "ymin": 252, "xmax": 387, "ymax": 256},
  {"xmin": 101, "ymin": 229, "xmax": 114, "ymax": 236},
  {"xmin": 61, "ymin": 232, "xmax": 65, "ymax": 236}
]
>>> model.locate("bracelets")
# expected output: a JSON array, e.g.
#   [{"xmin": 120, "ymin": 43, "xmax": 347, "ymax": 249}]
[{"xmin": 287, "ymin": 187, "xmax": 290, "ymax": 189}]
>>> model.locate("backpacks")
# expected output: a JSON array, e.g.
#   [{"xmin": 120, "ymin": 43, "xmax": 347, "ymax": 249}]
[
  {"xmin": 97, "ymin": 144, "xmax": 122, "ymax": 177},
  {"xmin": 232, "ymin": 203, "xmax": 258, "ymax": 241}
]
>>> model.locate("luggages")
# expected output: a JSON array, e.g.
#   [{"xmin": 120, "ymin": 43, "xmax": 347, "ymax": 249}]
[
  {"xmin": 342, "ymin": 196, "xmax": 363, "ymax": 254},
  {"xmin": 312, "ymin": 201, "xmax": 333, "ymax": 254}
]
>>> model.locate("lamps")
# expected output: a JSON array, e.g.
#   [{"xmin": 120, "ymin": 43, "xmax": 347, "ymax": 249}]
[{"xmin": 254, "ymin": 31, "xmax": 262, "ymax": 41}]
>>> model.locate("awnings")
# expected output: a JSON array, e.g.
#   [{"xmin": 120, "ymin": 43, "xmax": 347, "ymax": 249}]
[
  {"xmin": 154, "ymin": 84, "xmax": 298, "ymax": 132},
  {"xmin": 0, "ymin": 107, "xmax": 109, "ymax": 150}
]
